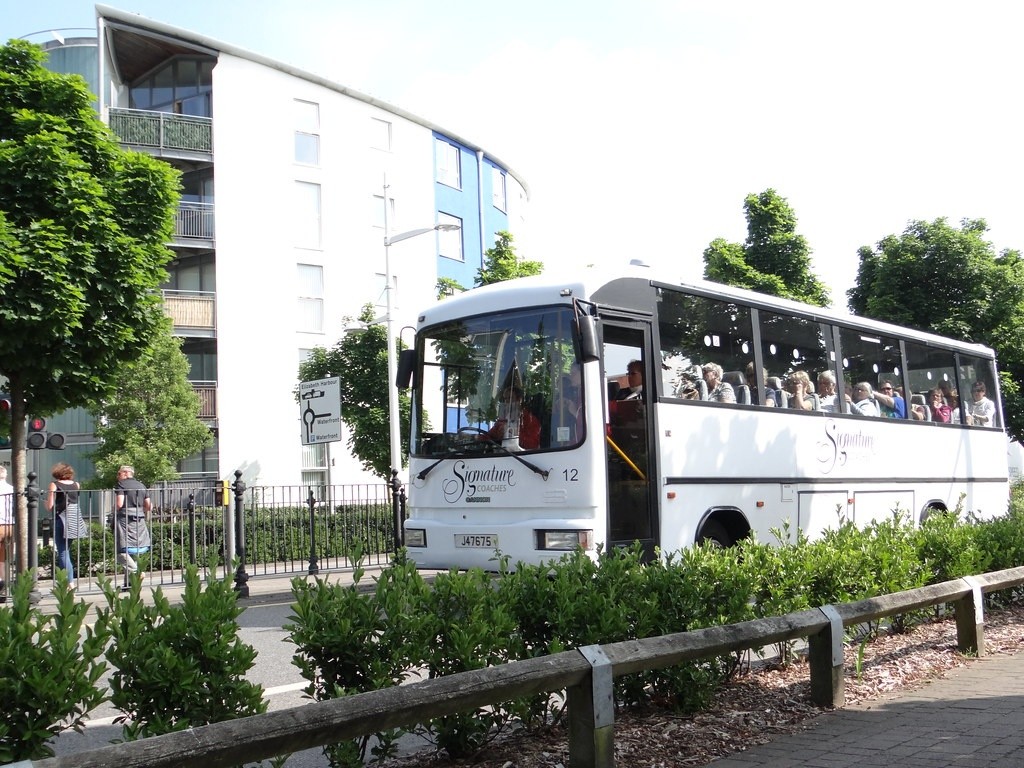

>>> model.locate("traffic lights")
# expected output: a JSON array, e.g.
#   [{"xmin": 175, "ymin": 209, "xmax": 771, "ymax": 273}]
[
  {"xmin": 0, "ymin": 431, "xmax": 14, "ymax": 448},
  {"xmin": 27, "ymin": 414, "xmax": 47, "ymax": 431},
  {"xmin": 26, "ymin": 431, "xmax": 66, "ymax": 449},
  {"xmin": 1, "ymin": 392, "xmax": 36, "ymax": 413}
]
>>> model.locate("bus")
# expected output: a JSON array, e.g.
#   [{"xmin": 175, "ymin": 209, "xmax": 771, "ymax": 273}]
[{"xmin": 402, "ymin": 265, "xmax": 1011, "ymax": 574}]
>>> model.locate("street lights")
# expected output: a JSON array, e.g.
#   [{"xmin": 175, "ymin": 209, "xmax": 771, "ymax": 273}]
[{"xmin": 383, "ymin": 172, "xmax": 460, "ymax": 513}]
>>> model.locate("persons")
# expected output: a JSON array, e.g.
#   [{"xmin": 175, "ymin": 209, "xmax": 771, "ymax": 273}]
[
  {"xmin": 556, "ymin": 362, "xmax": 584, "ymax": 446},
  {"xmin": 615, "ymin": 361, "xmax": 643, "ymax": 400},
  {"xmin": 109, "ymin": 465, "xmax": 151, "ymax": 591},
  {"xmin": 44, "ymin": 462, "xmax": 80, "ymax": 590},
  {"xmin": 681, "ymin": 362, "xmax": 997, "ymax": 428},
  {"xmin": 0, "ymin": 465, "xmax": 14, "ymax": 603},
  {"xmin": 458, "ymin": 386, "xmax": 541, "ymax": 450}
]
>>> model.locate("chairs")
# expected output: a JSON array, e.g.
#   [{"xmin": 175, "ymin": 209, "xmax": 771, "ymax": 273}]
[{"xmin": 526, "ymin": 371, "xmax": 996, "ymax": 452}]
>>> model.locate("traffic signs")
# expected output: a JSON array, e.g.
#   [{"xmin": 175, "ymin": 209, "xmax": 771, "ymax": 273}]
[{"xmin": 298, "ymin": 375, "xmax": 342, "ymax": 446}]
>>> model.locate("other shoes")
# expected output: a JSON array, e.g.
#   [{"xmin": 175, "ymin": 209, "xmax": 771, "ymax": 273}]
[
  {"xmin": 138, "ymin": 570, "xmax": 145, "ymax": 588},
  {"xmin": 66, "ymin": 582, "xmax": 75, "ymax": 594},
  {"xmin": 121, "ymin": 582, "xmax": 132, "ymax": 592}
]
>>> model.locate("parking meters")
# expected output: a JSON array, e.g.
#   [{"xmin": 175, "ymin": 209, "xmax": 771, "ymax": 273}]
[{"xmin": 214, "ymin": 480, "xmax": 229, "ymax": 508}]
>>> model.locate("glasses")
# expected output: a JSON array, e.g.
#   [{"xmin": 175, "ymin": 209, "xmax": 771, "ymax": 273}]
[
  {"xmin": 118, "ymin": 473, "xmax": 122, "ymax": 476},
  {"xmin": 626, "ymin": 372, "xmax": 639, "ymax": 376},
  {"xmin": 498, "ymin": 396, "xmax": 512, "ymax": 403},
  {"xmin": 880, "ymin": 387, "xmax": 893, "ymax": 391},
  {"xmin": 971, "ymin": 389, "xmax": 981, "ymax": 393}
]
